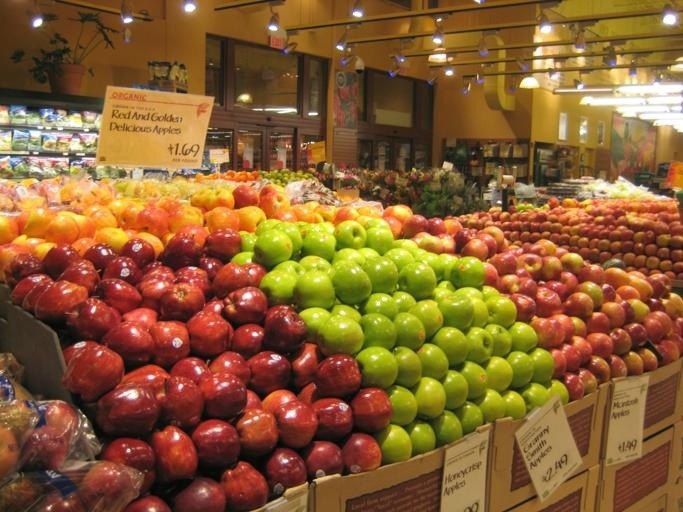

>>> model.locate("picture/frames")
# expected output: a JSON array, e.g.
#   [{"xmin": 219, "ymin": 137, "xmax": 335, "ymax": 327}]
[{"xmin": 608, "ymin": 109, "xmax": 658, "ymax": 184}]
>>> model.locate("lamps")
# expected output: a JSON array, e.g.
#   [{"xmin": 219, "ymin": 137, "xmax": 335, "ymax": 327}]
[
  {"xmin": 605, "ymin": 46, "xmax": 623, "ymax": 70},
  {"xmin": 554, "ymin": 80, "xmax": 683, "ymax": 133},
  {"xmin": 335, "ymin": 3, "xmax": 365, "ymax": 66},
  {"xmin": 574, "ymin": 28, "xmax": 591, "ymax": 56},
  {"xmin": 509, "ymin": 56, "xmax": 562, "ymax": 94},
  {"xmin": 534, "ymin": 5, "xmax": 555, "ymax": 37},
  {"xmin": 184, "ymin": 0, "xmax": 197, "ymax": 15},
  {"xmin": 653, "ymin": 70, "xmax": 664, "ymax": 85},
  {"xmin": 660, "ymin": 2, "xmax": 683, "ymax": 29},
  {"xmin": 424, "ymin": 13, "xmax": 457, "ymax": 86},
  {"xmin": 387, "ymin": 51, "xmax": 407, "ymax": 79},
  {"xmin": 27, "ymin": 9, "xmax": 45, "ymax": 29},
  {"xmin": 460, "ymin": 44, "xmax": 492, "ymax": 95},
  {"xmin": 119, "ymin": 4, "xmax": 133, "ymax": 24},
  {"xmin": 269, "ymin": 0, "xmax": 298, "ymax": 54},
  {"xmin": 628, "ymin": 60, "xmax": 642, "ymax": 79}
]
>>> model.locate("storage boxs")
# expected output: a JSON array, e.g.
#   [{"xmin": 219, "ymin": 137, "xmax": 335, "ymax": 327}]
[
  {"xmin": 490, "ymin": 360, "xmax": 683, "ymax": 512},
  {"xmin": 310, "ymin": 424, "xmax": 492, "ymax": 511},
  {"xmin": 255, "ymin": 482, "xmax": 309, "ymax": 511},
  {"xmin": 148, "ymin": 79, "xmax": 189, "ymax": 94}
]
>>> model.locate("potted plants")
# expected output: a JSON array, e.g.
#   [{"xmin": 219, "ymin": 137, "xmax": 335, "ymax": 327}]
[{"xmin": 9, "ymin": 12, "xmax": 119, "ymax": 95}]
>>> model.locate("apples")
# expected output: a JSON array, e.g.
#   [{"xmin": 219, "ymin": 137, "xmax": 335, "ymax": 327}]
[{"xmin": 0, "ymin": 175, "xmax": 683, "ymax": 512}]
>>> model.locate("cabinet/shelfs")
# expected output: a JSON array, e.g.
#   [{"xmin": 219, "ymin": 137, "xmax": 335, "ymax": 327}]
[{"xmin": 0, "ymin": 85, "xmax": 163, "ymax": 181}]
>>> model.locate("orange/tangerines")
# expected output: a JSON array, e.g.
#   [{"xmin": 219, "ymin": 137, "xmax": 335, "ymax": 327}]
[{"xmin": 260, "ymin": 169, "xmax": 314, "ymax": 186}]
